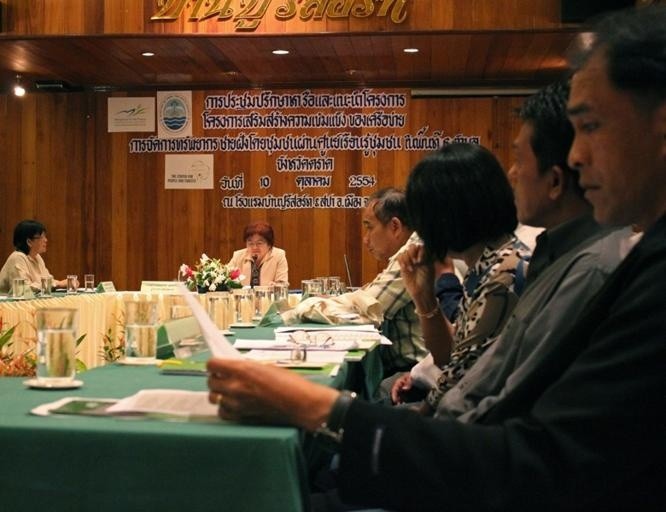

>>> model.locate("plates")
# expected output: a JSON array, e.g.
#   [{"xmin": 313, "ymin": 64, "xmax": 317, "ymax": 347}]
[
  {"xmin": 119, "ymin": 358, "xmax": 162, "ymax": 365},
  {"xmin": 230, "ymin": 324, "xmax": 257, "ymax": 329},
  {"xmin": 24, "ymin": 380, "xmax": 84, "ymax": 389},
  {"xmin": 221, "ymin": 330, "xmax": 236, "ymax": 336}
]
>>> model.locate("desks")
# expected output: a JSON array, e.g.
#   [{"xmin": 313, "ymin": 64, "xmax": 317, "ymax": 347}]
[
  {"xmin": 0, "ymin": 296, "xmax": 399, "ymax": 512},
  {"xmin": 0, "ymin": 282, "xmax": 280, "ymax": 377}
]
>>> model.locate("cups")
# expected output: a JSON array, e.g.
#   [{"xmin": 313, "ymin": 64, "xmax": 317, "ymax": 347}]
[
  {"xmin": 67, "ymin": 274, "xmax": 78, "ymax": 294},
  {"xmin": 233, "ymin": 289, "xmax": 255, "ymax": 325},
  {"xmin": 123, "ymin": 292, "xmax": 157, "ymax": 361},
  {"xmin": 273, "ymin": 283, "xmax": 289, "ymax": 303},
  {"xmin": 84, "ymin": 274, "xmax": 95, "ymax": 291},
  {"xmin": 41, "ymin": 276, "xmax": 52, "ymax": 296},
  {"xmin": 12, "ymin": 278, "xmax": 24, "ymax": 298},
  {"xmin": 205, "ymin": 291, "xmax": 230, "ymax": 329},
  {"xmin": 301, "ymin": 276, "xmax": 343, "ymax": 297},
  {"xmin": 35, "ymin": 306, "xmax": 76, "ymax": 386},
  {"xmin": 253, "ymin": 286, "xmax": 270, "ymax": 319}
]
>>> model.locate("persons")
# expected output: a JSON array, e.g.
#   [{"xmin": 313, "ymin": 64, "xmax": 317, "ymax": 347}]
[
  {"xmin": 336, "ymin": 188, "xmax": 438, "ymax": 403},
  {"xmin": 222, "ymin": 221, "xmax": 289, "ymax": 290},
  {"xmin": 433, "ymin": 254, "xmax": 470, "ymax": 325},
  {"xmin": 391, "ymin": 142, "xmax": 534, "ymax": 414},
  {"xmin": 207, "ymin": 6, "xmax": 666, "ymax": 512},
  {"xmin": 390, "ymin": 81, "xmax": 646, "ymax": 421},
  {"xmin": 0, "ymin": 220, "xmax": 79, "ymax": 294}
]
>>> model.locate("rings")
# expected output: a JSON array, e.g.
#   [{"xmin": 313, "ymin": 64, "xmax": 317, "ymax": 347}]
[{"xmin": 214, "ymin": 393, "xmax": 225, "ymax": 405}]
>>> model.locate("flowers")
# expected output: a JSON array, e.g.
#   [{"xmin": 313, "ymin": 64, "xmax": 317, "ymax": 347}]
[{"xmin": 177, "ymin": 251, "xmax": 247, "ymax": 296}]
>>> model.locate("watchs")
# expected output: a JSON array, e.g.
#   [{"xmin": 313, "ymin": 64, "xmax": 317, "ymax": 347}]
[{"xmin": 311, "ymin": 389, "xmax": 358, "ymax": 453}]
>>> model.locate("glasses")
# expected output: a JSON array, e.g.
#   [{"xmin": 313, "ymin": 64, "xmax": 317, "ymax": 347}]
[
  {"xmin": 29, "ymin": 233, "xmax": 47, "ymax": 240},
  {"xmin": 249, "ymin": 239, "xmax": 268, "ymax": 247}
]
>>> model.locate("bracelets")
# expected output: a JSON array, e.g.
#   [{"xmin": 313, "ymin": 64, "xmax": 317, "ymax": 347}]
[{"xmin": 414, "ymin": 296, "xmax": 440, "ymax": 319}]
